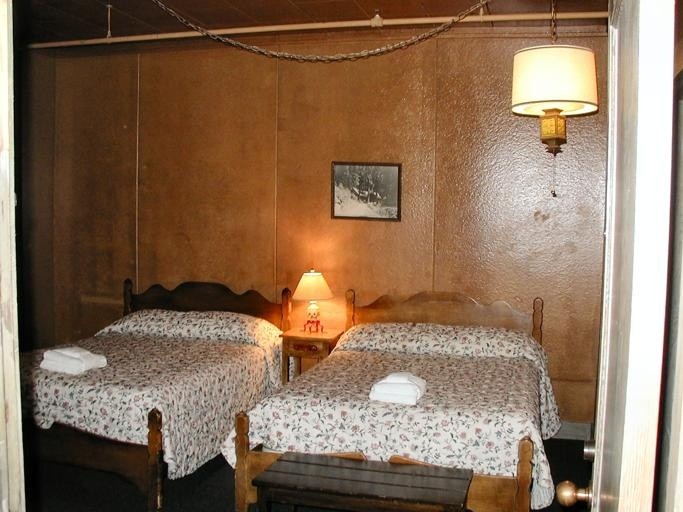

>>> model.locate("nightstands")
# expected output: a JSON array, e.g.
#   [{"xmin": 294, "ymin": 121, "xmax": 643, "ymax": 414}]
[{"xmin": 281, "ymin": 331, "xmax": 341, "ymax": 379}]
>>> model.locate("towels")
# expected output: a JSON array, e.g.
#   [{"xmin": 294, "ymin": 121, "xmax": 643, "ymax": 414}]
[
  {"xmin": 368, "ymin": 371, "xmax": 427, "ymax": 408},
  {"xmin": 38, "ymin": 345, "xmax": 108, "ymax": 376}
]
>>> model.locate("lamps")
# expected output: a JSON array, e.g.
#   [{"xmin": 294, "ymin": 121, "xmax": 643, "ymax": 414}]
[
  {"xmin": 291, "ymin": 269, "xmax": 333, "ymax": 330},
  {"xmin": 511, "ymin": 3, "xmax": 599, "ymax": 197},
  {"xmin": 370, "ymin": 11, "xmax": 382, "ymax": 29}
]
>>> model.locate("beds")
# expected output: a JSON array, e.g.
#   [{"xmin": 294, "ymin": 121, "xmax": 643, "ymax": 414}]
[
  {"xmin": 221, "ymin": 288, "xmax": 556, "ymax": 512},
  {"xmin": 23, "ymin": 279, "xmax": 281, "ymax": 512}
]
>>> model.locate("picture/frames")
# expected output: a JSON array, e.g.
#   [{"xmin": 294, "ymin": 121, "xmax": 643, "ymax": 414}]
[{"xmin": 329, "ymin": 159, "xmax": 402, "ymax": 222}]
